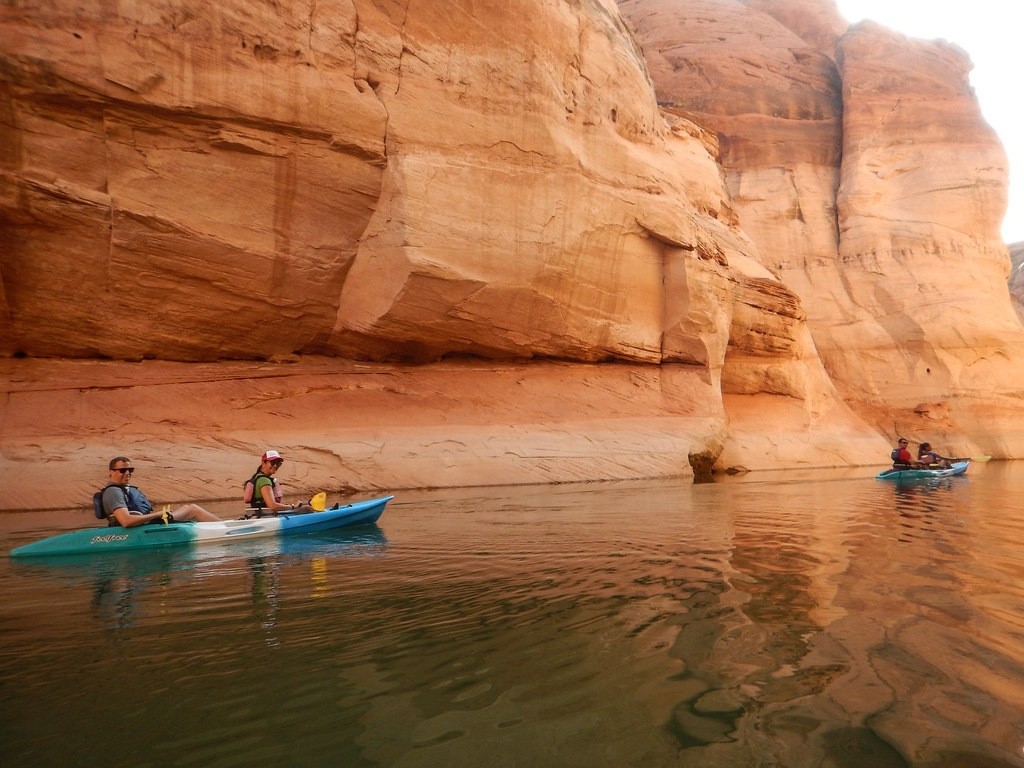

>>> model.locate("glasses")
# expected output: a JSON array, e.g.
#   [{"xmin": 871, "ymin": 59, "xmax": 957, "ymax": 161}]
[
  {"xmin": 901, "ymin": 442, "xmax": 909, "ymax": 445},
  {"xmin": 112, "ymin": 467, "xmax": 135, "ymax": 473},
  {"xmin": 267, "ymin": 460, "xmax": 282, "ymax": 467}
]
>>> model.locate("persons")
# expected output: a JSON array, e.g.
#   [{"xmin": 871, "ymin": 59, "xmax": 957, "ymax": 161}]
[
  {"xmin": 250, "ymin": 450, "xmax": 339, "ymax": 518},
  {"xmin": 893, "ymin": 437, "xmax": 931, "ymax": 471},
  {"xmin": 918, "ymin": 443, "xmax": 953, "ymax": 470},
  {"xmin": 101, "ymin": 456, "xmax": 223, "ymax": 528}
]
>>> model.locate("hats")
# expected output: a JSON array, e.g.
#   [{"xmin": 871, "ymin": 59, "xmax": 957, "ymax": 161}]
[{"xmin": 262, "ymin": 451, "xmax": 284, "ymax": 462}]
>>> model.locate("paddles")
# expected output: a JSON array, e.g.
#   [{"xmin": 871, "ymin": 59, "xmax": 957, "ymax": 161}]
[
  {"xmin": 160, "ymin": 502, "xmax": 174, "ymax": 529},
  {"xmin": 924, "ymin": 453, "xmax": 993, "ymax": 469},
  {"xmin": 300, "ymin": 488, "xmax": 329, "ymax": 514}
]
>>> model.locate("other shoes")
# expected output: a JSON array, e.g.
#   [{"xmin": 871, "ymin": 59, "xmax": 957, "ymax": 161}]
[{"xmin": 334, "ymin": 503, "xmax": 339, "ymax": 510}]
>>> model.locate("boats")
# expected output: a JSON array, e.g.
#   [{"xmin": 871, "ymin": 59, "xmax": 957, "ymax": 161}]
[
  {"xmin": 874, "ymin": 460, "xmax": 970, "ymax": 480},
  {"xmin": 6, "ymin": 493, "xmax": 395, "ymax": 558}
]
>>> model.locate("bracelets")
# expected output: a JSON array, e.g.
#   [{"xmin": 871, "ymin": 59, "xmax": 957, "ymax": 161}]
[{"xmin": 292, "ymin": 504, "xmax": 295, "ymax": 510}]
